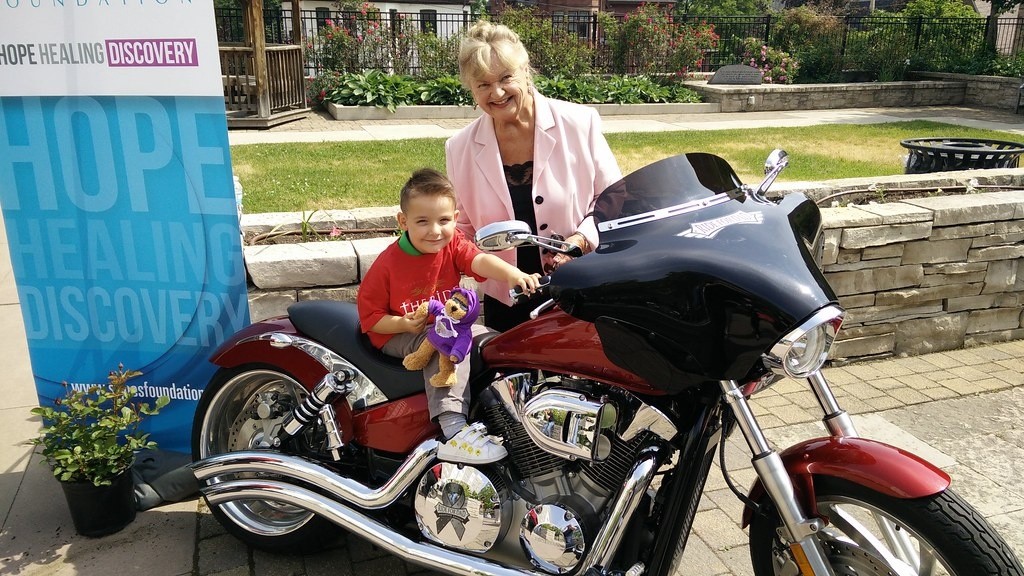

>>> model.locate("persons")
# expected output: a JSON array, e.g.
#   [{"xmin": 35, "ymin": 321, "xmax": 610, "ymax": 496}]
[
  {"xmin": 542, "ymin": 410, "xmax": 555, "ymax": 437},
  {"xmin": 357, "ymin": 168, "xmax": 542, "ymax": 464},
  {"xmin": 445, "ymin": 22, "xmax": 623, "ymax": 332}
]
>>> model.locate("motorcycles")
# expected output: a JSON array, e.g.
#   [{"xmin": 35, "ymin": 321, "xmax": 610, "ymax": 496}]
[{"xmin": 186, "ymin": 148, "xmax": 1024, "ymax": 576}]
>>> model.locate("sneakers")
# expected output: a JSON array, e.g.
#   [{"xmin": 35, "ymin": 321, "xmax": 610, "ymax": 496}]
[{"xmin": 436, "ymin": 420, "xmax": 508, "ymax": 464}]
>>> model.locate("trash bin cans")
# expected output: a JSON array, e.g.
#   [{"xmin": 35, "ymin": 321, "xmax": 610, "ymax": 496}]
[{"xmin": 898, "ymin": 138, "xmax": 1024, "ymax": 175}]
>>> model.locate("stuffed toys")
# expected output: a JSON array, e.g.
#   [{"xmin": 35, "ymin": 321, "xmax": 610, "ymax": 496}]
[{"xmin": 402, "ymin": 288, "xmax": 480, "ymax": 387}]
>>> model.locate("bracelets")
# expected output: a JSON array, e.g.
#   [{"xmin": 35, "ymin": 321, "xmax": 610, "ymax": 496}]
[{"xmin": 576, "ymin": 233, "xmax": 589, "ymax": 248}]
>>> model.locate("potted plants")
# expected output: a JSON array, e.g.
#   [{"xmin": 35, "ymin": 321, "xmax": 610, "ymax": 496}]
[{"xmin": 24, "ymin": 362, "xmax": 171, "ymax": 538}]
